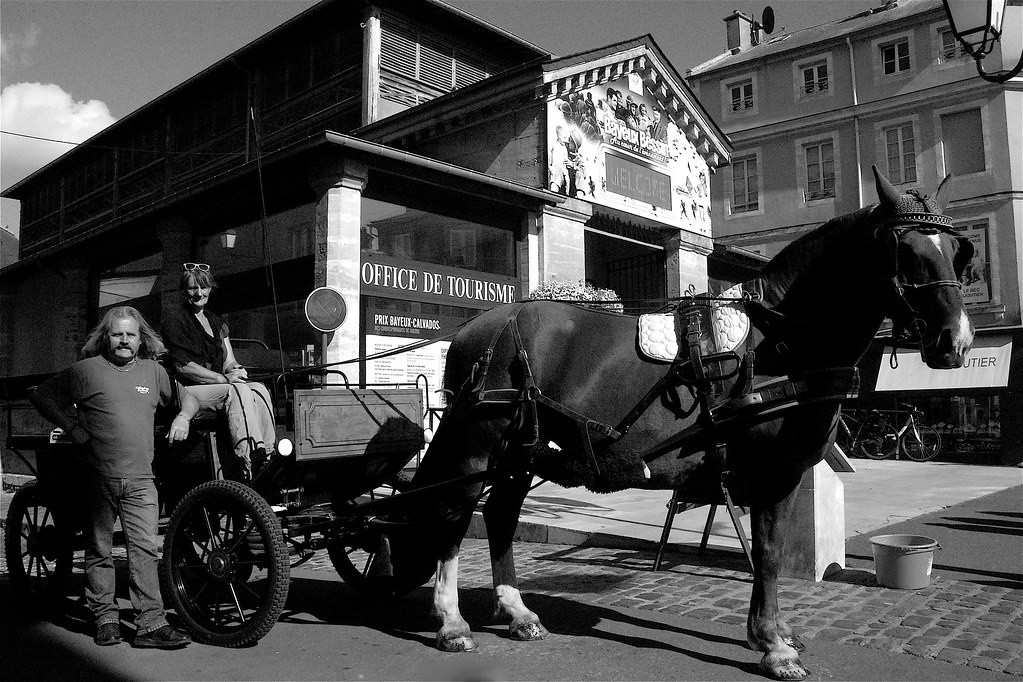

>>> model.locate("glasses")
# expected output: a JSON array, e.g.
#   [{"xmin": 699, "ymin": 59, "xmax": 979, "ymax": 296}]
[{"xmin": 183, "ymin": 263, "xmax": 210, "ymax": 271}]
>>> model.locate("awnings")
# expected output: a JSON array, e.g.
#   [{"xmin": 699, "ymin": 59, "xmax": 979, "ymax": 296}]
[{"xmin": 873, "ymin": 339, "xmax": 1013, "ymax": 392}]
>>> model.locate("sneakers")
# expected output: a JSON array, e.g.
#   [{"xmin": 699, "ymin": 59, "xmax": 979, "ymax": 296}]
[
  {"xmin": 134, "ymin": 625, "xmax": 191, "ymax": 647},
  {"xmin": 96, "ymin": 623, "xmax": 121, "ymax": 645}
]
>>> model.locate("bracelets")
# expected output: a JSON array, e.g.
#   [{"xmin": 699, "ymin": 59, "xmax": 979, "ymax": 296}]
[{"xmin": 178, "ymin": 413, "xmax": 191, "ymax": 421}]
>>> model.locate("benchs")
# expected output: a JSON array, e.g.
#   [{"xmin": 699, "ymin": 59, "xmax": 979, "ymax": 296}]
[{"xmin": 165, "ymin": 348, "xmax": 310, "ymax": 422}]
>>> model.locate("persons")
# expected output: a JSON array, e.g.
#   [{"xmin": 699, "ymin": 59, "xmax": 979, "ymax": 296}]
[
  {"xmin": 160, "ymin": 263, "xmax": 275, "ymax": 482},
  {"xmin": 28, "ymin": 306, "xmax": 201, "ymax": 649}
]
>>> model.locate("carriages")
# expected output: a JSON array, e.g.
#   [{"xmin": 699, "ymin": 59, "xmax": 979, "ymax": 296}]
[{"xmin": 4, "ymin": 164, "xmax": 977, "ymax": 675}]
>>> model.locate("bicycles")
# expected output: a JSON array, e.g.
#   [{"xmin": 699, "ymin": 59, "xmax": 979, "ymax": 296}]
[{"xmin": 838, "ymin": 400, "xmax": 942, "ymax": 463}]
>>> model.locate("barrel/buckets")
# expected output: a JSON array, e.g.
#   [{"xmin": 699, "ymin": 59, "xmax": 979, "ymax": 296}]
[{"xmin": 868, "ymin": 534, "xmax": 943, "ymax": 590}]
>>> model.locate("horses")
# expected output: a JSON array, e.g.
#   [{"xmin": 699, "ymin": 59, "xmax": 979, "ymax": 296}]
[{"xmin": 426, "ymin": 164, "xmax": 977, "ymax": 681}]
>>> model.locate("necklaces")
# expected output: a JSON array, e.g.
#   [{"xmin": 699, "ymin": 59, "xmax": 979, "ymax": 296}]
[{"xmin": 106, "ymin": 358, "xmax": 136, "ymax": 372}]
